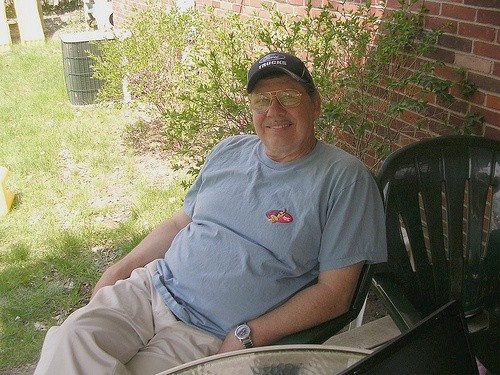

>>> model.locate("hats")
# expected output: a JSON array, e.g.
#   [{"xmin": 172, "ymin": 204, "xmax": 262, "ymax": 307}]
[{"xmin": 245, "ymin": 52, "xmax": 312, "ymax": 87}]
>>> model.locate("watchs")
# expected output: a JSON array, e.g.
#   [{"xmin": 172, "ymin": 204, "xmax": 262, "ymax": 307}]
[{"xmin": 235, "ymin": 322, "xmax": 254, "ymax": 348}]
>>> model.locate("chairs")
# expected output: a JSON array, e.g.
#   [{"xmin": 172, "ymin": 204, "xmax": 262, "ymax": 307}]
[{"xmin": 267, "ymin": 136, "xmax": 500, "ymax": 375}]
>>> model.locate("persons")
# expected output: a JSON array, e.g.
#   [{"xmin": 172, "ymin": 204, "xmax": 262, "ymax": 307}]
[{"xmin": 33, "ymin": 53, "xmax": 386, "ymax": 375}]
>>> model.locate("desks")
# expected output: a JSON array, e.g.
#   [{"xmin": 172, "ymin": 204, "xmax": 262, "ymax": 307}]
[{"xmin": 155, "ymin": 345, "xmax": 373, "ymax": 375}]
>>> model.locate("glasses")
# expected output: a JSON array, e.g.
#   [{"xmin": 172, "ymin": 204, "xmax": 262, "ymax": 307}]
[{"xmin": 249, "ymin": 89, "xmax": 312, "ymax": 111}]
